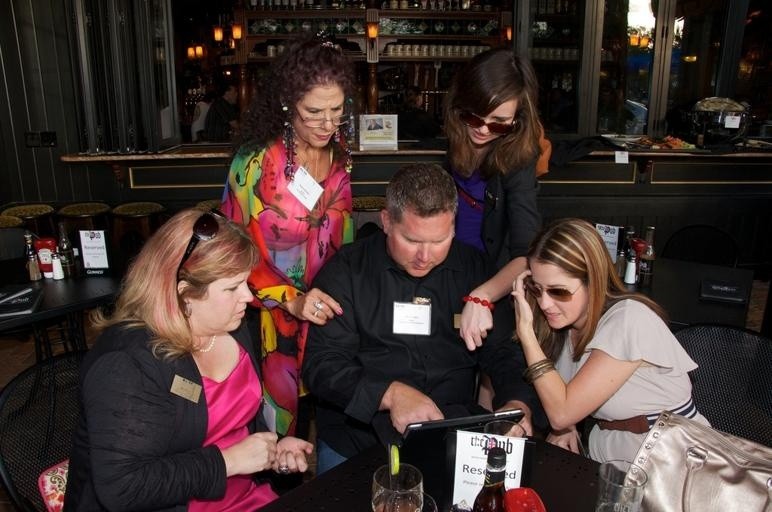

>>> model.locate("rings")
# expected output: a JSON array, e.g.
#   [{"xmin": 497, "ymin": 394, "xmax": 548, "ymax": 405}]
[
  {"xmin": 313, "ymin": 301, "xmax": 323, "ymax": 310},
  {"xmin": 278, "ymin": 464, "xmax": 289, "ymax": 473},
  {"xmin": 314, "ymin": 310, "xmax": 320, "ymax": 316}
]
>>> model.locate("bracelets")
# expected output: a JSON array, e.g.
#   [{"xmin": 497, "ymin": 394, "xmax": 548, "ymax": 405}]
[
  {"xmin": 460, "ymin": 295, "xmax": 494, "ymax": 309},
  {"xmin": 523, "ymin": 358, "xmax": 555, "ymax": 383}
]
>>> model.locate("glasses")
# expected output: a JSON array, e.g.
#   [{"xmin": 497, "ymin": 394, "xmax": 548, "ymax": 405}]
[
  {"xmin": 293, "ymin": 104, "xmax": 350, "ymax": 128},
  {"xmin": 458, "ymin": 108, "xmax": 514, "ymax": 137},
  {"xmin": 176, "ymin": 208, "xmax": 225, "ymax": 283},
  {"xmin": 526, "ymin": 280, "xmax": 587, "ymax": 302}
]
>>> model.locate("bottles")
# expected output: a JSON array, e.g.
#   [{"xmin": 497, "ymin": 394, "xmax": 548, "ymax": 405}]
[
  {"xmin": 28, "ymin": 253, "xmax": 42, "ymax": 281},
  {"xmin": 636, "ymin": 224, "xmax": 656, "ymax": 287},
  {"xmin": 57, "ymin": 220, "xmax": 78, "ymax": 267},
  {"xmin": 616, "ymin": 225, "xmax": 640, "ymax": 279},
  {"xmin": 50, "ymin": 253, "xmax": 65, "ymax": 280},
  {"xmin": 473, "ymin": 446, "xmax": 507, "ymax": 512},
  {"xmin": 623, "ymin": 254, "xmax": 636, "ymax": 283},
  {"xmin": 22, "ymin": 234, "xmax": 43, "ymax": 282}
]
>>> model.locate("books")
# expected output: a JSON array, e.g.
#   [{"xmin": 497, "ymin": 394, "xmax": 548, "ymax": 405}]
[
  {"xmin": 698, "ymin": 276, "xmax": 748, "ymax": 304},
  {"xmin": 0, "ymin": 282, "xmax": 45, "ymax": 319}
]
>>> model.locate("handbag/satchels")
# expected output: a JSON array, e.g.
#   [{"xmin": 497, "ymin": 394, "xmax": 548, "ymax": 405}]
[{"xmin": 614, "ymin": 408, "xmax": 772, "ymax": 512}]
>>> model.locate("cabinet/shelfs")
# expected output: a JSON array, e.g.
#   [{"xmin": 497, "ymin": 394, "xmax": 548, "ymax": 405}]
[{"xmin": 234, "ymin": 9, "xmax": 507, "ymax": 137}]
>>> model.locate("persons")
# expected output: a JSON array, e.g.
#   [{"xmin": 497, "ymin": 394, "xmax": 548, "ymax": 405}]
[
  {"xmin": 509, "ymin": 216, "xmax": 712, "ymax": 476},
  {"xmin": 602, "ymin": 227, "xmax": 610, "ymax": 235},
  {"xmin": 64, "ymin": 206, "xmax": 314, "ymax": 512},
  {"xmin": 302, "ymin": 163, "xmax": 533, "ymax": 480},
  {"xmin": 443, "ymin": 48, "xmax": 542, "ymax": 412},
  {"xmin": 205, "ymin": 82, "xmax": 240, "ymax": 142},
  {"xmin": 366, "ymin": 119, "xmax": 383, "ymax": 129},
  {"xmin": 396, "ymin": 85, "xmax": 442, "ymax": 138},
  {"xmin": 219, "ymin": 41, "xmax": 364, "ymax": 442}
]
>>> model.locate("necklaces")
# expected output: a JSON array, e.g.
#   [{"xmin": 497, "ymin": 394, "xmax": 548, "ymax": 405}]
[{"xmin": 184, "ymin": 335, "xmax": 217, "ymax": 352}]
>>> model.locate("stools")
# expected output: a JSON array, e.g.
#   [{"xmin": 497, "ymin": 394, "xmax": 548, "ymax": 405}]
[
  {"xmin": 112, "ymin": 200, "xmax": 167, "ymax": 244},
  {"xmin": 352, "ymin": 195, "xmax": 386, "ymax": 235},
  {"xmin": 1, "ymin": 204, "xmax": 57, "ymax": 240},
  {"xmin": 197, "ymin": 199, "xmax": 224, "ymax": 214},
  {"xmin": 0, "ymin": 216, "xmax": 24, "ymax": 229},
  {"xmin": 57, "ymin": 202, "xmax": 111, "ymax": 246}
]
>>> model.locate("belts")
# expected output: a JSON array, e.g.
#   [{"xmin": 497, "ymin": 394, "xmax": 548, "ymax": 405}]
[
  {"xmin": 455, "ymin": 188, "xmax": 482, "ymax": 210},
  {"xmin": 592, "ymin": 398, "xmax": 699, "ymax": 434}
]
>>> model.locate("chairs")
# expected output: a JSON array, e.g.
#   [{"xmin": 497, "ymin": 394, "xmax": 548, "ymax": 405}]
[
  {"xmin": 660, "ymin": 223, "xmax": 738, "ymax": 268},
  {"xmin": 672, "ymin": 323, "xmax": 772, "ymax": 448},
  {"xmin": 0, "ymin": 350, "xmax": 93, "ymax": 512}
]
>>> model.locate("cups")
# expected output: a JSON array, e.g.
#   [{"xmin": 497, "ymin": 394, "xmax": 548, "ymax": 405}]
[
  {"xmin": 372, "ymin": 463, "xmax": 424, "ymax": 512},
  {"xmin": 484, "ymin": 420, "xmax": 527, "ymax": 439},
  {"xmin": 594, "ymin": 459, "xmax": 648, "ymax": 512}
]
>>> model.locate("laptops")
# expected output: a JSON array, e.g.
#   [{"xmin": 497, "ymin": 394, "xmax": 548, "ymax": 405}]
[{"xmin": 369, "ymin": 400, "xmax": 525, "ymax": 463}]
